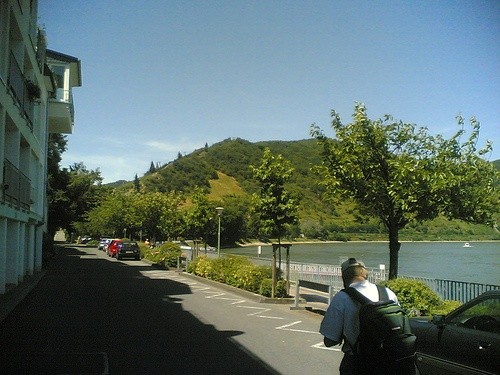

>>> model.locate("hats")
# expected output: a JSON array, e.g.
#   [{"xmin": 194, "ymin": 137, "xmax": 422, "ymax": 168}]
[{"xmin": 341, "ymin": 258, "xmax": 367, "ymax": 272}]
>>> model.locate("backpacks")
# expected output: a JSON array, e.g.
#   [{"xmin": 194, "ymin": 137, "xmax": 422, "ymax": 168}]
[{"xmin": 339, "ymin": 284, "xmax": 419, "ymax": 375}]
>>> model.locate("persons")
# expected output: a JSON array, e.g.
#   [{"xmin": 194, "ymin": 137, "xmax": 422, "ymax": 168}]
[
  {"xmin": 145, "ymin": 238, "xmax": 153, "ymax": 247},
  {"xmin": 319, "ymin": 257, "xmax": 401, "ymax": 375}
]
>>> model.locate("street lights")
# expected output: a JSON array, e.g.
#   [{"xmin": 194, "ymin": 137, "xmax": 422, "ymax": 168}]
[{"xmin": 215, "ymin": 206, "xmax": 225, "ymax": 258}]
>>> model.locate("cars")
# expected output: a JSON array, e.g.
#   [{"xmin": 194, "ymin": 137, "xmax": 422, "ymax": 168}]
[
  {"xmin": 97, "ymin": 237, "xmax": 141, "ymax": 260},
  {"xmin": 409, "ymin": 289, "xmax": 500, "ymax": 375}
]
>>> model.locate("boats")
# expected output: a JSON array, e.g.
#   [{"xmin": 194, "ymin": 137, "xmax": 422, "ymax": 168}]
[{"xmin": 463, "ymin": 243, "xmax": 473, "ymax": 247}]
